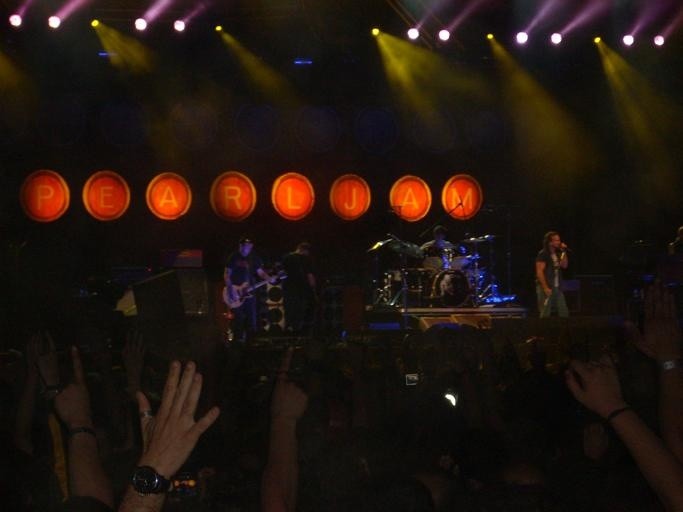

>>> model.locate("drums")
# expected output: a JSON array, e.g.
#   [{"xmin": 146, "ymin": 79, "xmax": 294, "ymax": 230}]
[
  {"xmin": 448, "ymin": 241, "xmax": 474, "ymax": 270},
  {"xmin": 422, "ymin": 243, "xmax": 449, "ymax": 270},
  {"xmin": 429, "ymin": 267, "xmax": 470, "ymax": 308},
  {"xmin": 402, "ymin": 268, "xmax": 430, "ymax": 292}
]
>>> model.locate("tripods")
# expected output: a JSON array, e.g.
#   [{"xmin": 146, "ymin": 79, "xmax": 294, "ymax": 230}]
[{"xmin": 478, "ymin": 264, "xmax": 506, "ymax": 306}]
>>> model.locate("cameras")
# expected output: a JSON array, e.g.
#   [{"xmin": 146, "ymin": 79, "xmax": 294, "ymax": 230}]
[{"xmin": 405, "ymin": 373, "xmax": 419, "ymax": 386}]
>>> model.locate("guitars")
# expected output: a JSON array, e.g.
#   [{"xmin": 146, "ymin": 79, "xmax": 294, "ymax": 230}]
[{"xmin": 221, "ymin": 269, "xmax": 289, "ymax": 309}]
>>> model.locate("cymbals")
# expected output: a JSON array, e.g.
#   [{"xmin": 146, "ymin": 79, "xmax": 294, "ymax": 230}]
[
  {"xmin": 476, "ymin": 233, "xmax": 503, "ymax": 239},
  {"xmin": 367, "ymin": 238, "xmax": 393, "ymax": 253},
  {"xmin": 388, "ymin": 240, "xmax": 425, "ymax": 260},
  {"xmin": 461, "ymin": 235, "xmax": 487, "ymax": 243}
]
